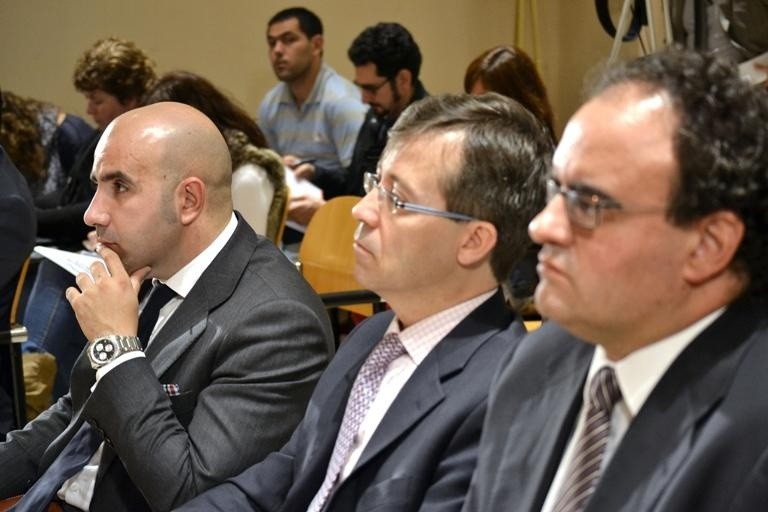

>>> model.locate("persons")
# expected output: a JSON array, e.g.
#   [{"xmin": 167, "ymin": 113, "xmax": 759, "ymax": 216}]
[
  {"xmin": 171, "ymin": 95, "xmax": 549, "ymax": 512},
  {"xmin": 1, "ymin": 37, "xmax": 286, "ymax": 404},
  {"xmin": 462, "ymin": 45, "xmax": 561, "ymax": 148},
  {"xmin": 1, "ymin": 100, "xmax": 334, "ymax": 512},
  {"xmin": 461, "ymin": 43, "xmax": 767, "ymax": 511},
  {"xmin": 258, "ymin": 10, "xmax": 371, "ymax": 168},
  {"xmin": 281, "ymin": 21, "xmax": 433, "ymax": 227}
]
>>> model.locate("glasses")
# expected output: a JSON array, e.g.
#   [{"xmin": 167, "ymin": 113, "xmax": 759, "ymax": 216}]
[
  {"xmin": 544, "ymin": 176, "xmax": 661, "ymax": 229},
  {"xmin": 362, "ymin": 172, "xmax": 471, "ymax": 223}
]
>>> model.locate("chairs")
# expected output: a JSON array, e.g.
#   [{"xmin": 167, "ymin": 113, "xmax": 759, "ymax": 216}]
[
  {"xmin": 12, "ymin": 254, "xmax": 87, "ymax": 385},
  {"xmin": 298, "ymin": 194, "xmax": 380, "ymax": 346}
]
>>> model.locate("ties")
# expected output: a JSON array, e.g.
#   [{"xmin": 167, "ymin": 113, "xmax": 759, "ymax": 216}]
[
  {"xmin": 540, "ymin": 368, "xmax": 620, "ymax": 512},
  {"xmin": 306, "ymin": 335, "xmax": 404, "ymax": 512},
  {"xmin": 4, "ymin": 285, "xmax": 177, "ymax": 512}
]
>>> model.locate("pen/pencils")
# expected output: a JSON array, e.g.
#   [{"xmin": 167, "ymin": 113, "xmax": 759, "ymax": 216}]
[{"xmin": 291, "ymin": 160, "xmax": 315, "ymax": 168}]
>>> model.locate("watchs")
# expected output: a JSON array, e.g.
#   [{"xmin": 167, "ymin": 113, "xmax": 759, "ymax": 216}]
[{"xmin": 86, "ymin": 335, "xmax": 143, "ymax": 368}]
